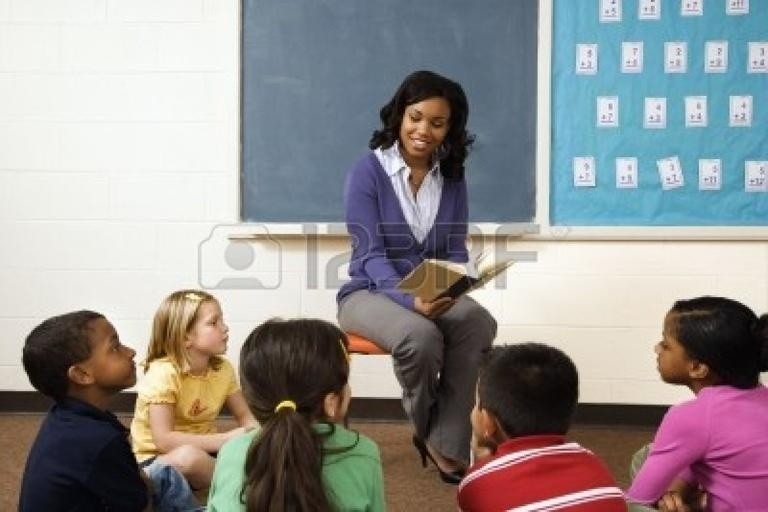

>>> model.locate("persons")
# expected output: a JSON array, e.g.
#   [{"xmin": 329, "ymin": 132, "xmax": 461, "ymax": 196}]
[
  {"xmin": 625, "ymin": 298, "xmax": 768, "ymax": 512},
  {"xmin": 17, "ymin": 311, "xmax": 195, "ymax": 512},
  {"xmin": 336, "ymin": 71, "xmax": 497, "ymax": 484},
  {"xmin": 130, "ymin": 291, "xmax": 258, "ymax": 492},
  {"xmin": 456, "ymin": 343, "xmax": 628, "ymax": 512},
  {"xmin": 206, "ymin": 321, "xmax": 386, "ymax": 512}
]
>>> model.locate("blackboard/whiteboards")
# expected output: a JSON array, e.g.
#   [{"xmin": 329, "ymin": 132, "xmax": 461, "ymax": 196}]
[{"xmin": 236, "ymin": 0, "xmax": 552, "ymax": 239}]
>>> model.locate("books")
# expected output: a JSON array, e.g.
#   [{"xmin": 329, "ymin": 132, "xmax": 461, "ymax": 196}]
[{"xmin": 393, "ymin": 250, "xmax": 516, "ymax": 303}]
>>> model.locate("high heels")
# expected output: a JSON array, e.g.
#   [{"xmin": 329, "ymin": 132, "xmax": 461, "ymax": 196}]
[{"xmin": 413, "ymin": 433, "xmax": 465, "ymax": 484}]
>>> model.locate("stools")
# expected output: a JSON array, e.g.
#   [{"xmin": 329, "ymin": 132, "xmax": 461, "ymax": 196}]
[{"xmin": 341, "ymin": 331, "xmax": 390, "ymax": 430}]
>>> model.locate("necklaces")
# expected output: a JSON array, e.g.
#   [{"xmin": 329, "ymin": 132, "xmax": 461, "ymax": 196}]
[{"xmin": 408, "ymin": 176, "xmax": 424, "ymax": 192}]
